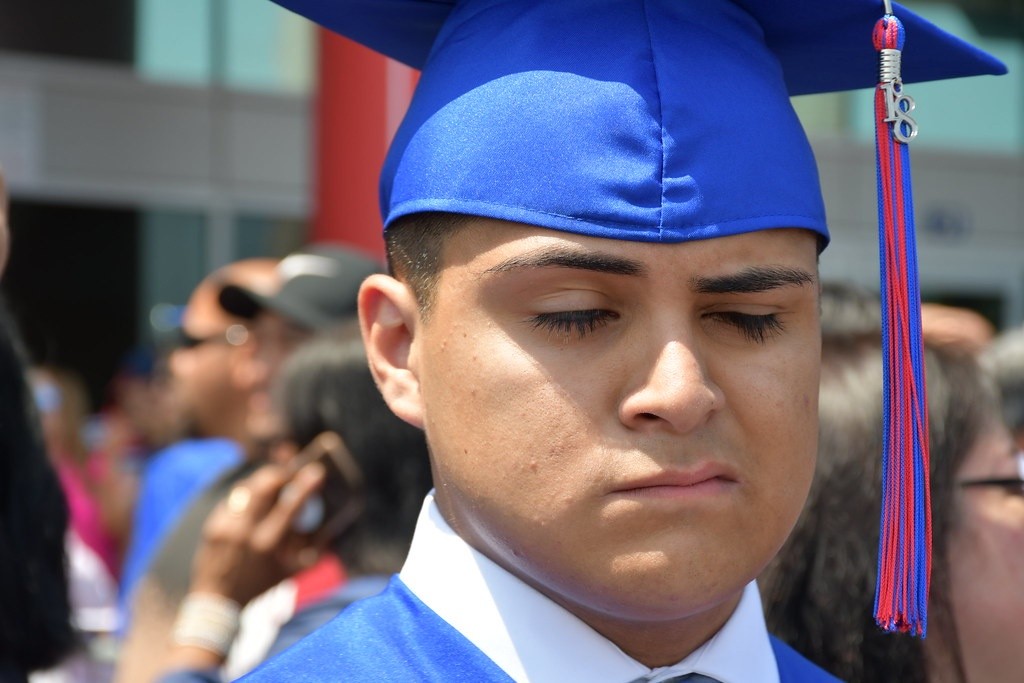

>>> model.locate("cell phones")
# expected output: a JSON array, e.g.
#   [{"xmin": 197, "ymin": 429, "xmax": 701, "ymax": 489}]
[{"xmin": 252, "ymin": 431, "xmax": 367, "ymax": 552}]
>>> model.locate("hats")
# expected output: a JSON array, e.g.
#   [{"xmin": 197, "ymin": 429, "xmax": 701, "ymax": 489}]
[{"xmin": 268, "ymin": 0, "xmax": 1012, "ymax": 640}]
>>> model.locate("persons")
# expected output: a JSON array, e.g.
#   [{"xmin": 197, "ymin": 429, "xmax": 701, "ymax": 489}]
[
  {"xmin": 757, "ymin": 276, "xmax": 1024, "ymax": 683},
  {"xmin": 229, "ymin": 0, "xmax": 1009, "ymax": 683},
  {"xmin": 0, "ymin": 249, "xmax": 433, "ymax": 683}
]
